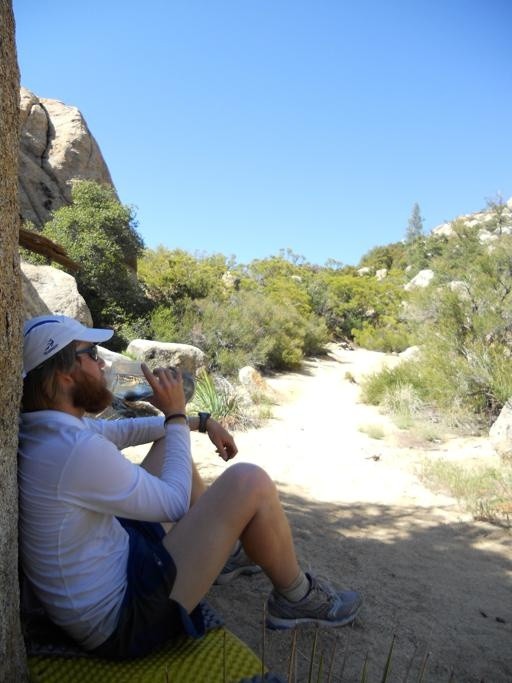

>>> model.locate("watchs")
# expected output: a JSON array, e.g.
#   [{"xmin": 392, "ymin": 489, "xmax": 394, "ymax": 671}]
[{"xmin": 198, "ymin": 412, "xmax": 211, "ymax": 433}]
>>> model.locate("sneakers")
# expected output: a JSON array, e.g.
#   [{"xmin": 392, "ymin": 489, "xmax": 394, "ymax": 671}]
[
  {"xmin": 212, "ymin": 540, "xmax": 265, "ymax": 585},
  {"xmin": 263, "ymin": 572, "xmax": 364, "ymax": 631}
]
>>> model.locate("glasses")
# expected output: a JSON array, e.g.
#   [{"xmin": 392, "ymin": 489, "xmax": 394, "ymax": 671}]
[{"xmin": 75, "ymin": 342, "xmax": 99, "ymax": 362}]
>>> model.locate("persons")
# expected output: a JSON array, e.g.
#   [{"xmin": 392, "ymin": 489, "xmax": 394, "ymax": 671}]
[{"xmin": 16, "ymin": 315, "xmax": 363, "ymax": 663}]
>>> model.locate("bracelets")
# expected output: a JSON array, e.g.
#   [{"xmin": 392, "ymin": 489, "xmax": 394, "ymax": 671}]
[{"xmin": 163, "ymin": 414, "xmax": 188, "ymax": 429}]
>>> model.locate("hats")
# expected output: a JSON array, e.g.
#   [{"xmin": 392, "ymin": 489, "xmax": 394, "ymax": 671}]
[{"xmin": 22, "ymin": 314, "xmax": 115, "ymax": 377}]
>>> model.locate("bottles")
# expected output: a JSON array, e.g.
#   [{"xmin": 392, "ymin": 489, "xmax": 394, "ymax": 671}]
[{"xmin": 102, "ymin": 359, "xmax": 196, "ymax": 408}]
